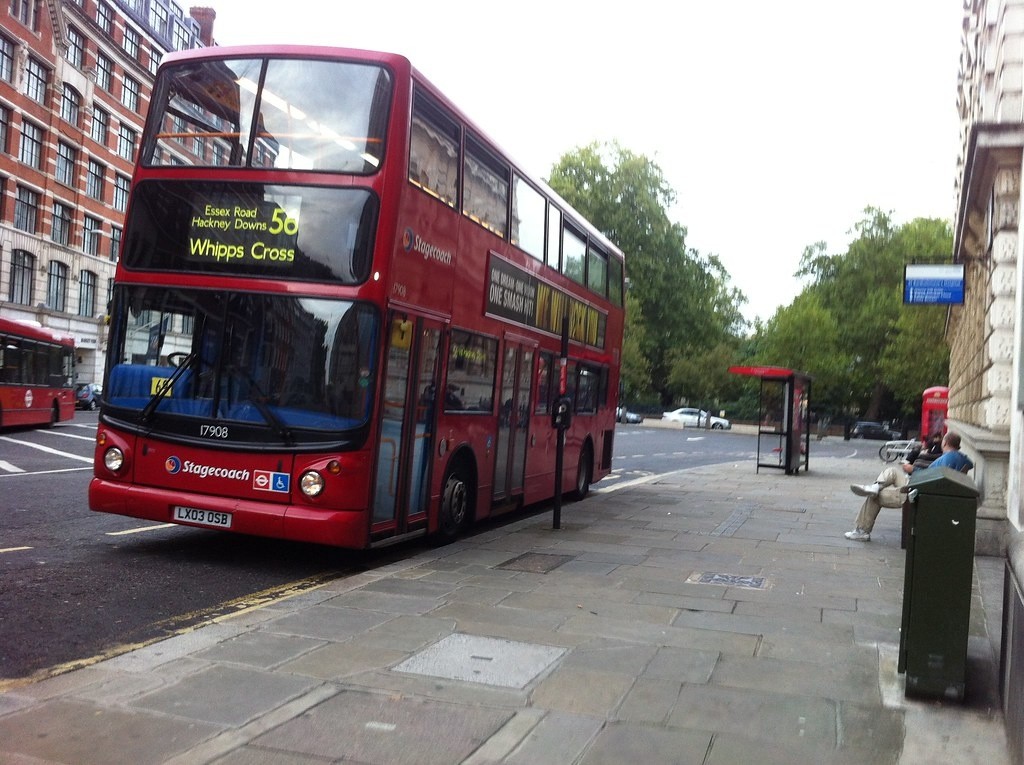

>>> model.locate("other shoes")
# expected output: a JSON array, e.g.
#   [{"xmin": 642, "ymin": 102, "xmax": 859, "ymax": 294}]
[
  {"xmin": 850, "ymin": 483, "xmax": 881, "ymax": 499},
  {"xmin": 844, "ymin": 527, "xmax": 870, "ymax": 540}
]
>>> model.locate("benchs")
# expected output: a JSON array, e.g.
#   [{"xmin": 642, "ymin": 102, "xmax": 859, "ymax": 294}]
[{"xmin": 902, "ymin": 464, "xmax": 972, "ymax": 549}]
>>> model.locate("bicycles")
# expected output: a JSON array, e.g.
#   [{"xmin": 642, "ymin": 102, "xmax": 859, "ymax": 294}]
[{"xmin": 879, "ymin": 438, "xmax": 917, "ymax": 461}]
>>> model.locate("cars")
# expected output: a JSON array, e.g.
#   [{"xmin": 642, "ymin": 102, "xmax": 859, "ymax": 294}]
[
  {"xmin": 661, "ymin": 408, "xmax": 732, "ymax": 430},
  {"xmin": 615, "ymin": 406, "xmax": 642, "ymax": 423},
  {"xmin": 849, "ymin": 421, "xmax": 902, "ymax": 439},
  {"xmin": 73, "ymin": 381, "xmax": 103, "ymax": 411}
]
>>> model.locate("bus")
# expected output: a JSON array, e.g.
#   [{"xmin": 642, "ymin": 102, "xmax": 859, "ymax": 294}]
[
  {"xmin": 0, "ymin": 317, "xmax": 75, "ymax": 429},
  {"xmin": 87, "ymin": 44, "xmax": 628, "ymax": 549}
]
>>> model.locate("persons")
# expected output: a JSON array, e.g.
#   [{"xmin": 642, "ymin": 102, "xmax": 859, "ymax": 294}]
[{"xmin": 845, "ymin": 433, "xmax": 973, "ymax": 540}]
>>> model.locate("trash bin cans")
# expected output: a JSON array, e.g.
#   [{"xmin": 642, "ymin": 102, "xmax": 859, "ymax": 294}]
[{"xmin": 898, "ymin": 467, "xmax": 980, "ymax": 704}]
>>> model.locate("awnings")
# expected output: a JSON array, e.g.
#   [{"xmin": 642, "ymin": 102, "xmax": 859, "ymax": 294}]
[{"xmin": 726, "ymin": 365, "xmax": 792, "ymax": 376}]
